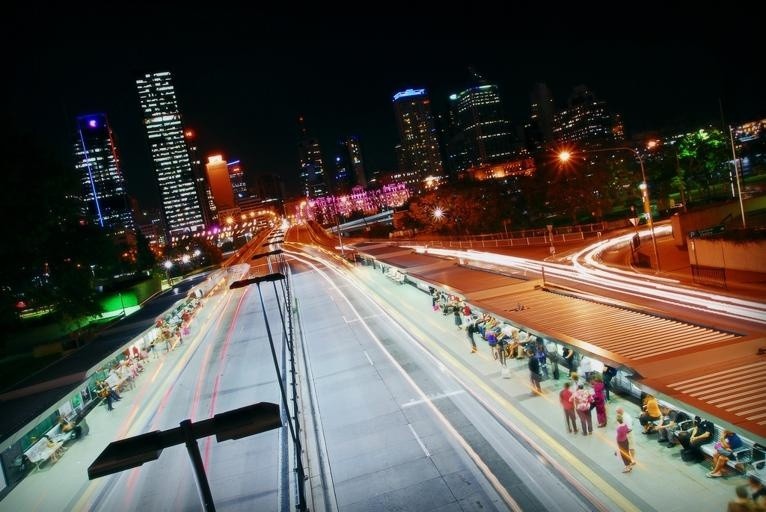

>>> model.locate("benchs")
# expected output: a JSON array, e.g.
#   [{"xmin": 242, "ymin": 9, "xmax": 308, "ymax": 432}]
[
  {"xmin": 654, "ymin": 399, "xmax": 766, "ymax": 483},
  {"xmin": 23, "ymin": 422, "xmax": 73, "ymax": 472},
  {"xmin": 103, "ymin": 366, "xmax": 134, "ymax": 393}
]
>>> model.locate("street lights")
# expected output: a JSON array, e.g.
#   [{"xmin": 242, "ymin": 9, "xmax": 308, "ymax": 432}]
[
  {"xmin": 251, "ymin": 248, "xmax": 292, "ymax": 353},
  {"xmin": 557, "ymin": 145, "xmax": 662, "ymax": 275},
  {"xmin": 85, "ymin": 400, "xmax": 284, "ymax": 512},
  {"xmin": 230, "ymin": 272, "xmax": 300, "ymax": 447}
]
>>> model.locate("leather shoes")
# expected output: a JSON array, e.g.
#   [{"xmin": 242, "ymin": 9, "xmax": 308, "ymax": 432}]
[
  {"xmin": 667, "ymin": 443, "xmax": 676, "ymax": 448},
  {"xmin": 658, "ymin": 439, "xmax": 667, "ymax": 442},
  {"xmin": 597, "ymin": 422, "xmax": 606, "ymax": 427}
]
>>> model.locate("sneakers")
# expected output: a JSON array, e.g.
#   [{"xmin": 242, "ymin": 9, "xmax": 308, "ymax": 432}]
[{"xmin": 706, "ymin": 471, "xmax": 722, "ymax": 478}]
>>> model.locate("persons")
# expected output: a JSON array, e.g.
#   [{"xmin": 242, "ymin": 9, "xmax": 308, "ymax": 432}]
[
  {"xmin": 93, "ymin": 297, "xmax": 203, "ymax": 412},
  {"xmin": 639, "ymin": 392, "xmax": 715, "ymax": 464},
  {"xmin": 705, "ymin": 429, "xmax": 743, "ymax": 478},
  {"xmin": 431, "ymin": 291, "xmax": 559, "ymax": 395},
  {"xmin": 727, "ymin": 474, "xmax": 766, "ymax": 511},
  {"xmin": 614, "ymin": 406, "xmax": 636, "ymax": 473},
  {"xmin": 559, "ymin": 346, "xmax": 618, "ymax": 436}
]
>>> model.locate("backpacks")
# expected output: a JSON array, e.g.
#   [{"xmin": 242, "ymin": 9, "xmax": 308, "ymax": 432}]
[{"xmin": 578, "ymin": 398, "xmax": 590, "ymax": 411}]
